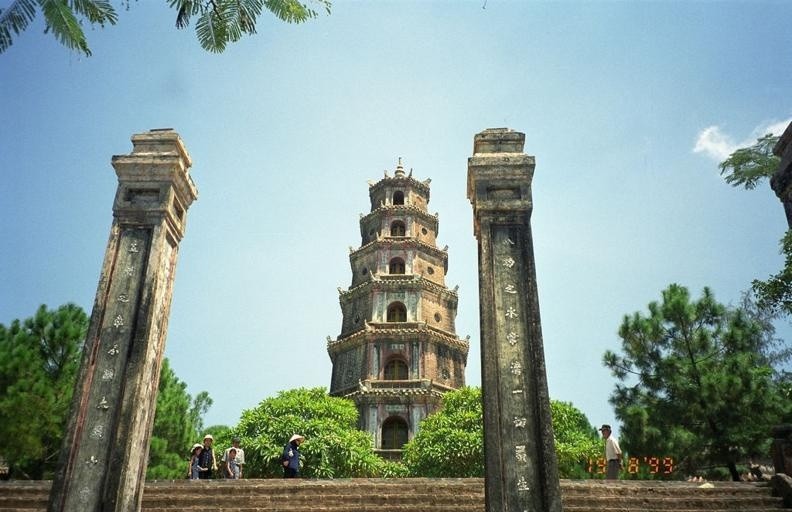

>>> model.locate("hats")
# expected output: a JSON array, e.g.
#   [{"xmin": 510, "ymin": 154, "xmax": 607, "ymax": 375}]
[
  {"xmin": 289, "ymin": 434, "xmax": 305, "ymax": 445},
  {"xmin": 204, "ymin": 434, "xmax": 213, "ymax": 441},
  {"xmin": 191, "ymin": 443, "xmax": 204, "ymax": 454}
]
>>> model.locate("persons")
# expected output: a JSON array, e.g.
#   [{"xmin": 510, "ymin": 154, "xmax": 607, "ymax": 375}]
[
  {"xmin": 224, "ymin": 449, "xmax": 243, "ymax": 479},
  {"xmin": 221, "ymin": 438, "xmax": 247, "ymax": 477},
  {"xmin": 187, "ymin": 443, "xmax": 210, "ymax": 479},
  {"xmin": 281, "ymin": 433, "xmax": 305, "ymax": 479},
  {"xmin": 198, "ymin": 435, "xmax": 218, "ymax": 479},
  {"xmin": 598, "ymin": 424, "xmax": 624, "ymax": 478}
]
